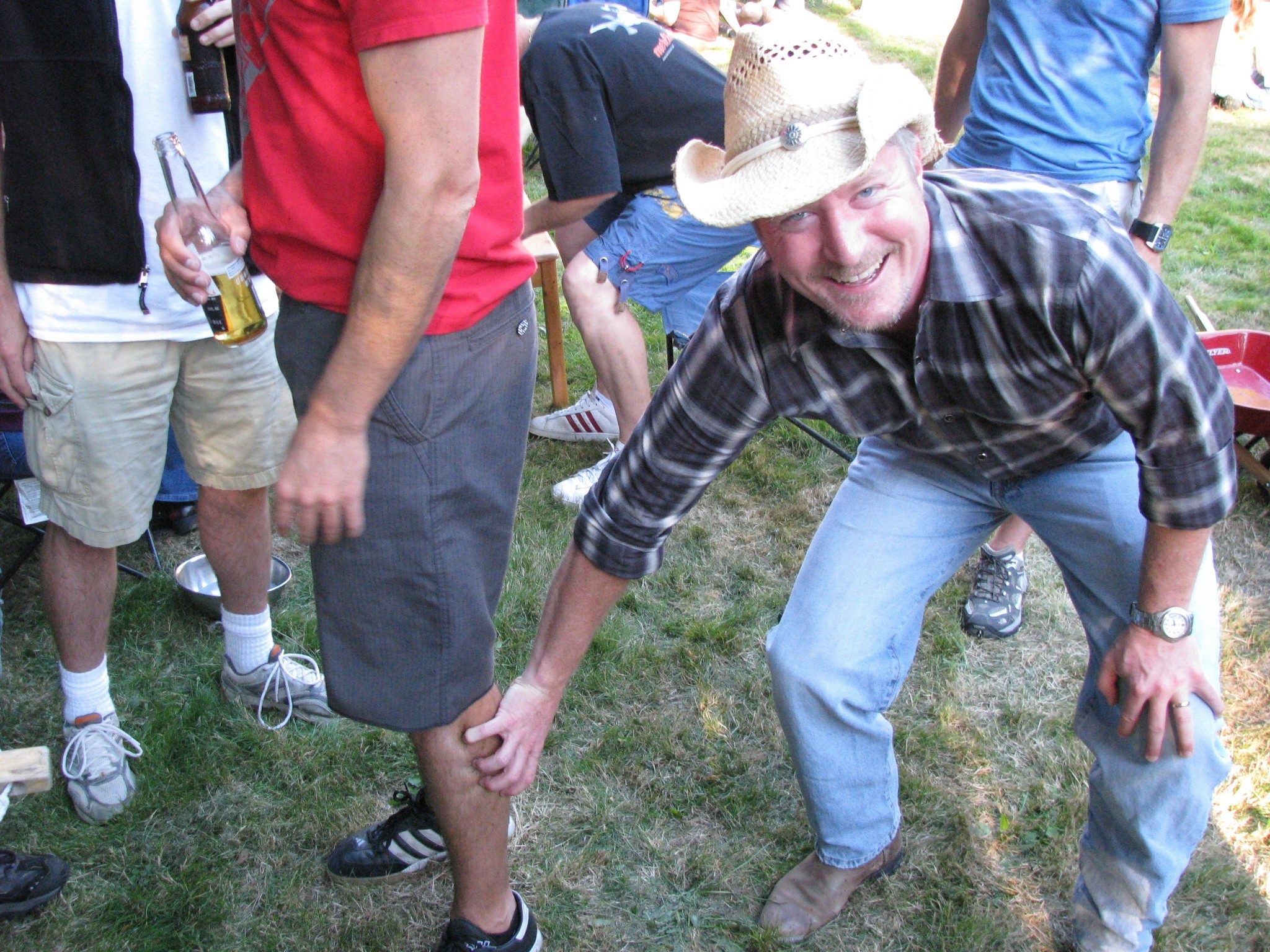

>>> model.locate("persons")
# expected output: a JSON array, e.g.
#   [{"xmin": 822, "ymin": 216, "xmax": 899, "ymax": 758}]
[
  {"xmin": 463, "ymin": 7, "xmax": 1237, "ymax": 952},
  {"xmin": 0, "ymin": 0, "xmax": 341, "ymax": 827},
  {"xmin": 516, "ymin": 0, "xmax": 761, "ymax": 506},
  {"xmin": 646, "ymin": 0, "xmax": 805, "ymax": 41},
  {"xmin": 933, "ymin": 0, "xmax": 1229, "ymax": 641},
  {"xmin": 154, "ymin": 0, "xmax": 542, "ymax": 952}
]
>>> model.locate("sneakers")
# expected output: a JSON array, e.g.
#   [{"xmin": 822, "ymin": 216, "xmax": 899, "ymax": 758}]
[
  {"xmin": 528, "ymin": 380, "xmax": 620, "ymax": 443},
  {"xmin": 551, "ymin": 437, "xmax": 626, "ymax": 507},
  {"xmin": 437, "ymin": 890, "xmax": 543, "ymax": 952},
  {"xmin": 62, "ymin": 710, "xmax": 144, "ymax": 827},
  {"xmin": 961, "ymin": 541, "xmax": 1028, "ymax": 637},
  {"xmin": 221, "ymin": 643, "xmax": 348, "ymax": 730},
  {"xmin": 0, "ymin": 850, "xmax": 71, "ymax": 915},
  {"xmin": 326, "ymin": 783, "xmax": 516, "ymax": 882}
]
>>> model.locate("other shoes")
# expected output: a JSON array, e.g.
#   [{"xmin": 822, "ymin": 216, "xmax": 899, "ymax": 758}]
[
  {"xmin": 153, "ymin": 498, "xmax": 200, "ymax": 536},
  {"xmin": 757, "ymin": 826, "xmax": 902, "ymax": 940}
]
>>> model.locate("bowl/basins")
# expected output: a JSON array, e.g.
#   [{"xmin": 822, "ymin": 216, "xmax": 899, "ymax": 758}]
[{"xmin": 175, "ymin": 554, "xmax": 293, "ymax": 619}]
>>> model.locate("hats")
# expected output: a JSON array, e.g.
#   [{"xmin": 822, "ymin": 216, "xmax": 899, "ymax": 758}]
[{"xmin": 671, "ymin": 7, "xmax": 936, "ymax": 228}]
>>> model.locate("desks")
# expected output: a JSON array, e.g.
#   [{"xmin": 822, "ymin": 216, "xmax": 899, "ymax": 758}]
[{"xmin": 521, "ymin": 192, "xmax": 568, "ymax": 411}]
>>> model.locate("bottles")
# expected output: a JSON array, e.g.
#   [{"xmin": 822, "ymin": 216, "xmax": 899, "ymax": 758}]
[
  {"xmin": 176, "ymin": 0, "xmax": 232, "ymax": 113},
  {"xmin": 154, "ymin": 130, "xmax": 267, "ymax": 349}
]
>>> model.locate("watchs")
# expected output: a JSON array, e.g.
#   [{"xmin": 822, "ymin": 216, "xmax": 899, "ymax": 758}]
[
  {"xmin": 1128, "ymin": 217, "xmax": 1173, "ymax": 253},
  {"xmin": 1130, "ymin": 601, "xmax": 1194, "ymax": 643}
]
